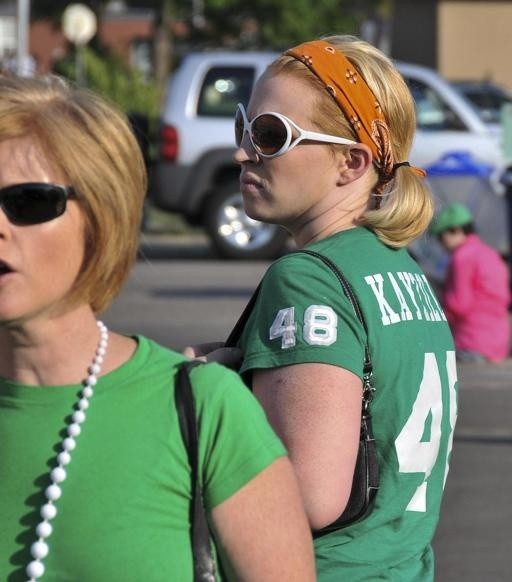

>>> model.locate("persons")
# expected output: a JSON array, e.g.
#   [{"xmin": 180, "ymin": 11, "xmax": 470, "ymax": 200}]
[
  {"xmin": 180, "ymin": 35, "xmax": 461, "ymax": 579},
  {"xmin": 428, "ymin": 199, "xmax": 510, "ymax": 367},
  {"xmin": 0, "ymin": 66, "xmax": 319, "ymax": 582}
]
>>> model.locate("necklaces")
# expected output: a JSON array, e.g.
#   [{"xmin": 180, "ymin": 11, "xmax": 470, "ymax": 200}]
[{"xmin": 24, "ymin": 320, "xmax": 111, "ymax": 582}]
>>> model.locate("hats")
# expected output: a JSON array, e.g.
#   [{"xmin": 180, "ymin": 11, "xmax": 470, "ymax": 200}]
[{"xmin": 426, "ymin": 202, "xmax": 476, "ymax": 236}]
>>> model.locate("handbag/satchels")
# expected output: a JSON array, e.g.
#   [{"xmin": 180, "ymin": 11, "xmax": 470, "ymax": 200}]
[{"xmin": 296, "ymin": 413, "xmax": 378, "ymax": 535}]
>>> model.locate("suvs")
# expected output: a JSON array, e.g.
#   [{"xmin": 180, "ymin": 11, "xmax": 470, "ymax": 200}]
[{"xmin": 127, "ymin": 50, "xmax": 512, "ymax": 259}]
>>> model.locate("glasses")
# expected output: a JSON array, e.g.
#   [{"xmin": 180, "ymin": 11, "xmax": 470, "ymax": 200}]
[
  {"xmin": 228, "ymin": 99, "xmax": 358, "ymax": 163},
  {"xmin": 0, "ymin": 180, "xmax": 82, "ymax": 229}
]
